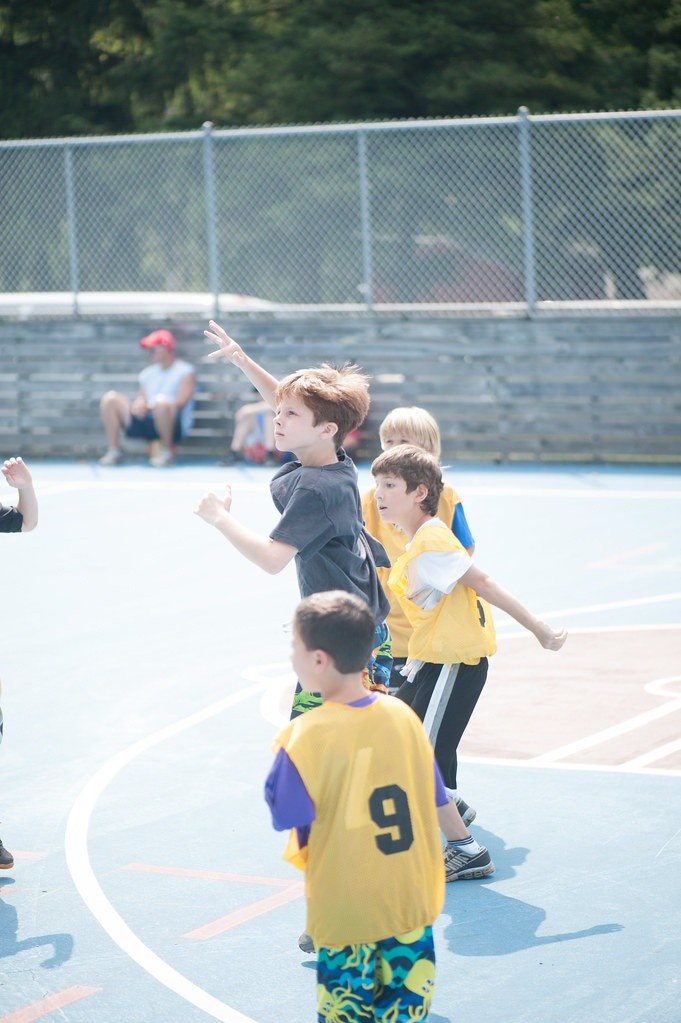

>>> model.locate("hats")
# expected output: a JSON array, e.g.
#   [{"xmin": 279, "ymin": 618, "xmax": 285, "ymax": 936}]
[{"xmin": 139, "ymin": 330, "xmax": 173, "ymax": 350}]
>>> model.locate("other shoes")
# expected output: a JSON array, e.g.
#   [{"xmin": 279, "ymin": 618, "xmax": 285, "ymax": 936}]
[
  {"xmin": 98, "ymin": 448, "xmax": 122, "ymax": 466},
  {"xmin": 0, "ymin": 840, "xmax": 12, "ymax": 869},
  {"xmin": 298, "ymin": 933, "xmax": 316, "ymax": 953},
  {"xmin": 149, "ymin": 450, "xmax": 172, "ymax": 466}
]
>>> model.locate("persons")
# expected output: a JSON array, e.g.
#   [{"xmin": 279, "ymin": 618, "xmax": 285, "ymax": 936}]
[
  {"xmin": 216, "ymin": 384, "xmax": 280, "ymax": 470},
  {"xmin": 366, "ymin": 228, "xmax": 681, "ymax": 301},
  {"xmin": 2, "ymin": 455, "xmax": 37, "ymax": 868},
  {"xmin": 264, "ymin": 590, "xmax": 453, "ymax": 1022},
  {"xmin": 191, "ymin": 318, "xmax": 389, "ymax": 957},
  {"xmin": 362, "ymin": 406, "xmax": 479, "ymax": 700},
  {"xmin": 100, "ymin": 329, "xmax": 196, "ymax": 466},
  {"xmin": 365, "ymin": 447, "xmax": 567, "ymax": 884}
]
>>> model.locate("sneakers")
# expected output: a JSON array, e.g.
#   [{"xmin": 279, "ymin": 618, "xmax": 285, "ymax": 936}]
[
  {"xmin": 443, "ymin": 845, "xmax": 495, "ymax": 883},
  {"xmin": 455, "ymin": 797, "xmax": 476, "ymax": 828}
]
